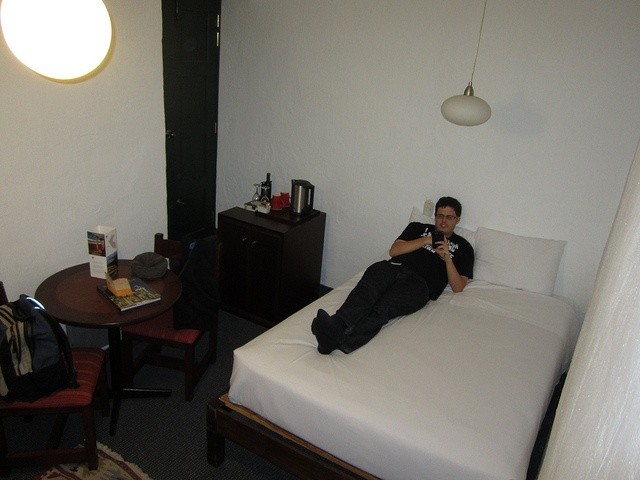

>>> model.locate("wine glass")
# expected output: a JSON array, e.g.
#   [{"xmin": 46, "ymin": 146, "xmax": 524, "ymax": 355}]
[
  {"xmin": 261, "ymin": 186, "xmax": 269, "ymax": 205},
  {"xmin": 252, "ymin": 184, "xmax": 260, "ymax": 204}
]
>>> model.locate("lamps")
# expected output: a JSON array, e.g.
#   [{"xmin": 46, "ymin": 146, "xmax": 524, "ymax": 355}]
[{"xmin": 441, "ymin": 2, "xmax": 492, "ymax": 125}]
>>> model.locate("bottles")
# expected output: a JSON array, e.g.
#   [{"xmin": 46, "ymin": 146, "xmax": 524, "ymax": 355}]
[
  {"xmin": 261, "ymin": 186, "xmax": 270, "ymax": 203},
  {"xmin": 264, "ymin": 172, "xmax": 272, "ymax": 200}
]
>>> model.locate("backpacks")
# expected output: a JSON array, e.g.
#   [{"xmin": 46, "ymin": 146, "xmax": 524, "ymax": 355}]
[{"xmin": 0, "ymin": 294, "xmax": 80, "ymax": 403}]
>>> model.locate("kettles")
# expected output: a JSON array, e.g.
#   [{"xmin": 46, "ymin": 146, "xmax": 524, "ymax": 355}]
[{"xmin": 291, "ymin": 179, "xmax": 315, "ymax": 216}]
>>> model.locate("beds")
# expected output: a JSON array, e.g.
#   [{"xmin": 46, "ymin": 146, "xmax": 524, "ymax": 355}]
[{"xmin": 206, "ymin": 208, "xmax": 578, "ymax": 480}]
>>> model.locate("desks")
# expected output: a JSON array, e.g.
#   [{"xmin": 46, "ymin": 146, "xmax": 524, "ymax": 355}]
[{"xmin": 34, "ymin": 260, "xmax": 182, "ymax": 436}]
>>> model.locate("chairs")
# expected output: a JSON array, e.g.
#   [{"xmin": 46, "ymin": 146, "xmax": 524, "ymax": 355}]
[
  {"xmin": 0, "ymin": 283, "xmax": 106, "ymax": 477},
  {"xmin": 122, "ymin": 232, "xmax": 223, "ymax": 400}
]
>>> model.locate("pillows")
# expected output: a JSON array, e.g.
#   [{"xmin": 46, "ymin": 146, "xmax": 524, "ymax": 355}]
[
  {"xmin": 410, "ymin": 208, "xmax": 474, "ymax": 247},
  {"xmin": 473, "ymin": 227, "xmax": 567, "ymax": 298}
]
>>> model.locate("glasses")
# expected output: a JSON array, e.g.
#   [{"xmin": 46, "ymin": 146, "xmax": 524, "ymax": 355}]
[{"xmin": 435, "ymin": 214, "xmax": 458, "ymax": 221}]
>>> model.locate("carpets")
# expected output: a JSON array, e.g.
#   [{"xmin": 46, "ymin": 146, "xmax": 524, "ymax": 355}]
[{"xmin": 28, "ymin": 439, "xmax": 151, "ymax": 480}]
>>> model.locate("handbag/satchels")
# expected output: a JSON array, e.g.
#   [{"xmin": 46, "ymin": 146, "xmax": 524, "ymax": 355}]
[{"xmin": 174, "ymin": 287, "xmax": 224, "ymax": 329}]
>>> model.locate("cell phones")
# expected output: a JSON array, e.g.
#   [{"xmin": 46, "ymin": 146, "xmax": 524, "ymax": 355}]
[{"xmin": 431, "ymin": 231, "xmax": 444, "ymax": 248}]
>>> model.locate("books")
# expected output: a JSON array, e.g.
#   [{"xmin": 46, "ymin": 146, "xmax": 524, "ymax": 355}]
[
  {"xmin": 96, "ymin": 276, "xmax": 162, "ymax": 313},
  {"xmin": 104, "ymin": 270, "xmax": 133, "ymax": 296}
]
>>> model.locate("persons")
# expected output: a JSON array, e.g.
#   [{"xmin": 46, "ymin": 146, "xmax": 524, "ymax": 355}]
[{"xmin": 312, "ymin": 197, "xmax": 474, "ymax": 354}]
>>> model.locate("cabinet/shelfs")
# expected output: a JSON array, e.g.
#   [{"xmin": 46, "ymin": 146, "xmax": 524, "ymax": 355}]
[{"xmin": 217, "ymin": 199, "xmax": 326, "ymax": 329}]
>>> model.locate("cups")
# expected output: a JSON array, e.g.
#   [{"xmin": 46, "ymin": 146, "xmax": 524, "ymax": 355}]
[
  {"xmin": 281, "ymin": 192, "xmax": 291, "ymax": 207},
  {"xmin": 270, "ymin": 194, "xmax": 285, "ymax": 211}
]
